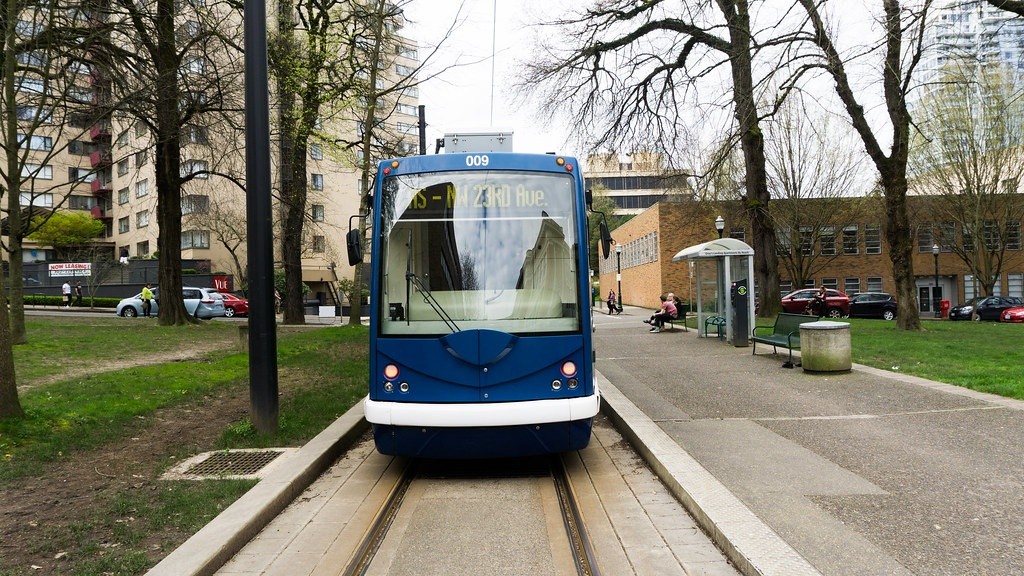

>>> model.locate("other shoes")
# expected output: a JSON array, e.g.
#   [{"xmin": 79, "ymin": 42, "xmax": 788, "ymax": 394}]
[
  {"xmin": 659, "ymin": 326, "xmax": 665, "ymax": 331},
  {"xmin": 650, "ymin": 328, "xmax": 655, "ymax": 331},
  {"xmin": 643, "ymin": 320, "xmax": 651, "ymax": 324},
  {"xmin": 650, "ymin": 322, "xmax": 656, "ymax": 326},
  {"xmin": 650, "ymin": 326, "xmax": 659, "ymax": 332}
]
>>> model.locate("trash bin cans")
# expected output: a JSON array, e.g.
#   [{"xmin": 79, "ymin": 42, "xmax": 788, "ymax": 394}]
[{"xmin": 799, "ymin": 321, "xmax": 852, "ymax": 374}]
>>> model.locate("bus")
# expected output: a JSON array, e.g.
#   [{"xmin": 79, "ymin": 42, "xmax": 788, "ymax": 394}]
[{"xmin": 346, "ymin": 130, "xmax": 614, "ymax": 476}]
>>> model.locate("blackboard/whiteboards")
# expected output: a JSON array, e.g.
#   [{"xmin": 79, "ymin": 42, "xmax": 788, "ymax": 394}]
[{"xmin": 319, "ymin": 306, "xmax": 335, "ymax": 318}]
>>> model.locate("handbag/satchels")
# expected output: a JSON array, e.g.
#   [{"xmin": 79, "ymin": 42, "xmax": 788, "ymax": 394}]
[
  {"xmin": 63, "ymin": 296, "xmax": 68, "ymax": 301},
  {"xmin": 140, "ymin": 302, "xmax": 148, "ymax": 308}
]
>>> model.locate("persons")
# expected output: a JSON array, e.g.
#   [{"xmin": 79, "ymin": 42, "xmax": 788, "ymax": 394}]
[
  {"xmin": 803, "ymin": 300, "xmax": 812, "ymax": 314},
  {"xmin": 607, "ymin": 289, "xmax": 620, "ymax": 315},
  {"xmin": 71, "ymin": 280, "xmax": 83, "ymax": 306},
  {"xmin": 643, "ymin": 293, "xmax": 681, "ymax": 332},
  {"xmin": 141, "ymin": 284, "xmax": 155, "ymax": 317},
  {"xmin": 275, "ymin": 287, "xmax": 280, "ymax": 314},
  {"xmin": 155, "ymin": 286, "xmax": 159, "ymax": 306},
  {"xmin": 815, "ymin": 286, "xmax": 827, "ymax": 318},
  {"xmin": 62, "ymin": 280, "xmax": 72, "ymax": 307}
]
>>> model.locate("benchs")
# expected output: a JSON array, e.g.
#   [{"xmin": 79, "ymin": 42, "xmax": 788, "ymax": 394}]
[
  {"xmin": 656, "ymin": 303, "xmax": 689, "ymax": 332},
  {"xmin": 705, "ymin": 315, "xmax": 726, "ymax": 340},
  {"xmin": 752, "ymin": 312, "xmax": 820, "ymax": 369}
]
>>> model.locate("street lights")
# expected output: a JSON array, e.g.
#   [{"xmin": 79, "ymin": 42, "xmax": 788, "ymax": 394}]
[
  {"xmin": 715, "ymin": 215, "xmax": 725, "ymax": 239},
  {"xmin": 616, "ymin": 243, "xmax": 623, "ymax": 313},
  {"xmin": 932, "ymin": 244, "xmax": 942, "ymax": 317}
]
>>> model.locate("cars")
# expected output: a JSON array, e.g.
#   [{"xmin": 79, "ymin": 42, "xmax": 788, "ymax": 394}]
[
  {"xmin": 848, "ymin": 293, "xmax": 897, "ymax": 321},
  {"xmin": 116, "ymin": 286, "xmax": 226, "ymax": 320},
  {"xmin": 950, "ymin": 296, "xmax": 1024, "ymax": 321},
  {"xmin": 1000, "ymin": 303, "xmax": 1024, "ymax": 323},
  {"xmin": 220, "ymin": 293, "xmax": 249, "ymax": 318},
  {"xmin": 781, "ymin": 288, "xmax": 851, "ymax": 319}
]
